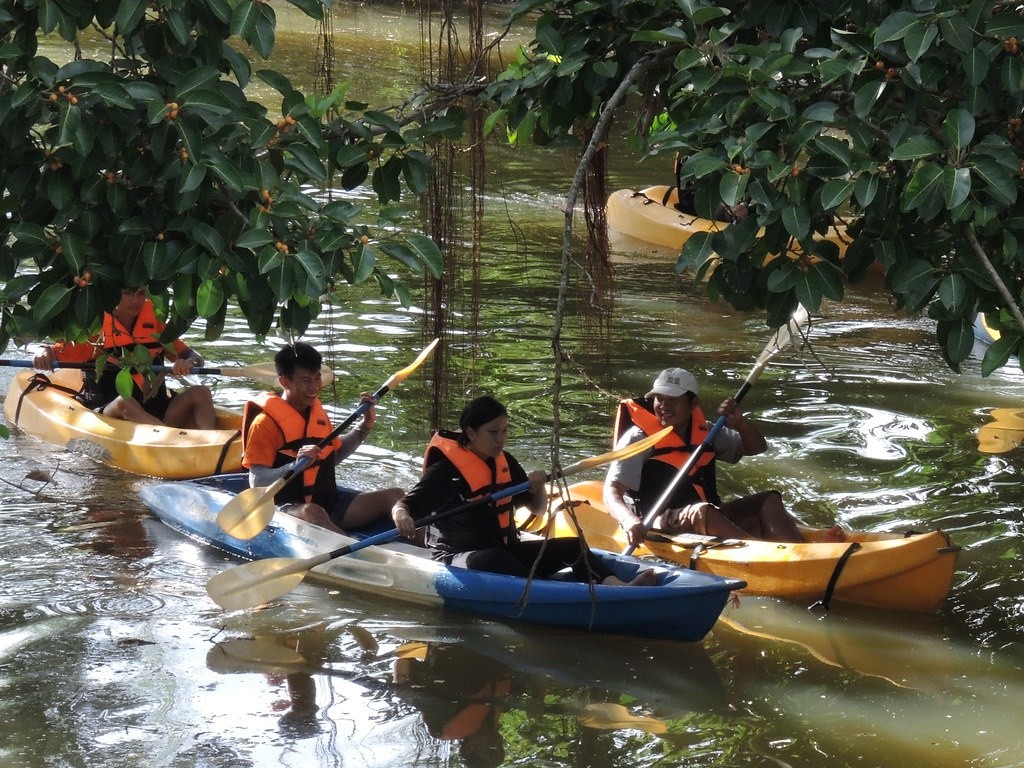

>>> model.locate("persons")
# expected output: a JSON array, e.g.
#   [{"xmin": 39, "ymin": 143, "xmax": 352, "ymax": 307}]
[
  {"xmin": 252, "ymin": 605, "xmax": 379, "ymax": 739},
  {"xmin": 601, "ymin": 367, "xmax": 846, "ymax": 550},
  {"xmin": 241, "ymin": 342, "xmax": 425, "ymax": 547},
  {"xmin": 392, "ymin": 617, "xmax": 514, "ymax": 768},
  {"xmin": 391, "ymin": 396, "xmax": 658, "ymax": 586},
  {"xmin": 34, "ymin": 288, "xmax": 218, "ymax": 430}
]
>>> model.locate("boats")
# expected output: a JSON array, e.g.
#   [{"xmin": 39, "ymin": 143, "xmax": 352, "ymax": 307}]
[
  {"xmin": 513, "ymin": 471, "xmax": 958, "ymax": 614},
  {"xmin": 7, "ymin": 358, "xmax": 260, "ymax": 479},
  {"xmin": 599, "ymin": 180, "xmax": 857, "ymax": 271},
  {"xmin": 132, "ymin": 466, "xmax": 749, "ymax": 638}
]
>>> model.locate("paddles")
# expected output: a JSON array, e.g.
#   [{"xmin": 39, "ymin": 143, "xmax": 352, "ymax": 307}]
[
  {"xmin": 215, "ymin": 336, "xmax": 440, "ymax": 540},
  {"xmin": 621, "ymin": 302, "xmax": 811, "ymax": 557},
  {"xmin": 0, "ymin": 359, "xmax": 334, "ymax": 390},
  {"xmin": 204, "ymin": 425, "xmax": 674, "ymax": 611}
]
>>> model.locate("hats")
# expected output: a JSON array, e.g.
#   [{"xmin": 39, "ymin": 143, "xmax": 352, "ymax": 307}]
[{"xmin": 644, "ymin": 366, "xmax": 700, "ymax": 399}]
